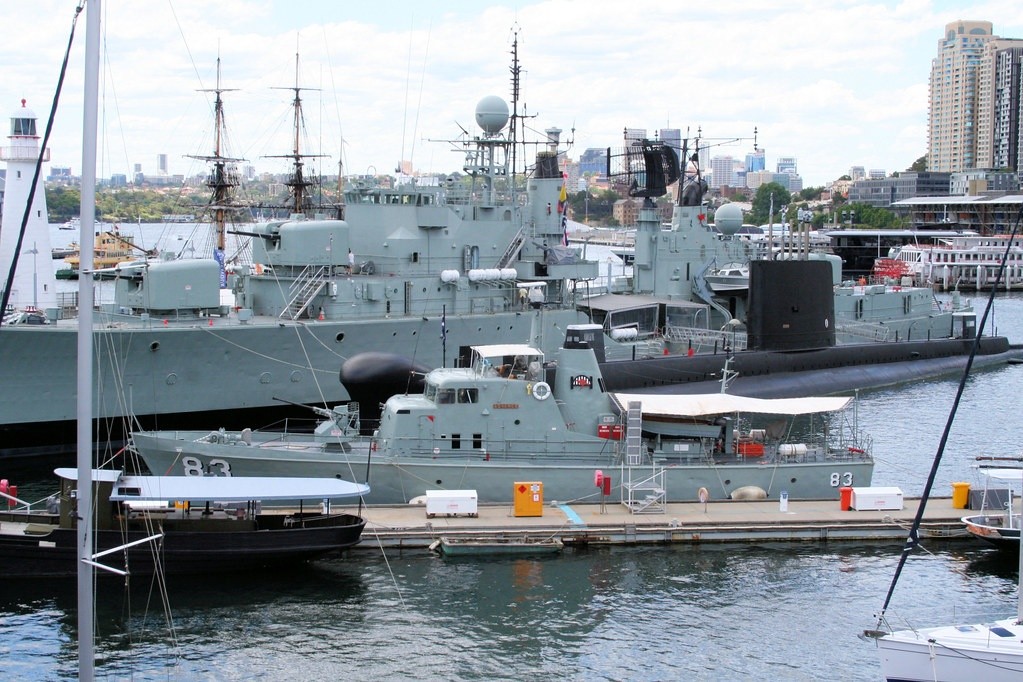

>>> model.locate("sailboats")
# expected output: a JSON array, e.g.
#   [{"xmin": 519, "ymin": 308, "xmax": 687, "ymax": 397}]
[{"xmin": 854, "ymin": 198, "xmax": 1023, "ymax": 682}]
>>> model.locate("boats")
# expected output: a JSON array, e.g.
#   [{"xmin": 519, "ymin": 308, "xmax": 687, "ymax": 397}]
[
  {"xmin": 0, "ymin": 435, "xmax": 374, "ymax": 572},
  {"xmin": 52, "ymin": 214, "xmax": 161, "ymax": 283},
  {"xmin": 126, "ymin": 298, "xmax": 875, "ymax": 512},
  {"xmin": 0, "ymin": 19, "xmax": 973, "ymax": 458},
  {"xmin": 609, "ymin": 193, "xmax": 1023, "ymax": 297},
  {"xmin": 959, "ymin": 464, "xmax": 1021, "ymax": 554}
]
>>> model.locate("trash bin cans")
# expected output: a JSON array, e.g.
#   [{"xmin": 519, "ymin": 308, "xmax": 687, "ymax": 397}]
[
  {"xmin": 951, "ymin": 482, "xmax": 971, "ymax": 509},
  {"xmin": 840, "ymin": 487, "xmax": 853, "ymax": 511}
]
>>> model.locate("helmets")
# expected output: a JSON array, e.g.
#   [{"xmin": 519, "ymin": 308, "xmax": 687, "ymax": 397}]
[{"xmin": 515, "ymin": 355, "xmax": 527, "ymax": 370}]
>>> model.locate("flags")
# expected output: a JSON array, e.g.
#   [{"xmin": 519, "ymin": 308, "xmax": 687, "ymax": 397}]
[{"xmin": 557, "ymin": 184, "xmax": 566, "ymax": 213}]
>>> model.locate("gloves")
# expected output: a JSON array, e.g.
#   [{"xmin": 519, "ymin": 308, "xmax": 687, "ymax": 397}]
[
  {"xmin": 510, "ymin": 374, "xmax": 516, "ymax": 379},
  {"xmin": 500, "ymin": 364, "xmax": 507, "ymax": 374}
]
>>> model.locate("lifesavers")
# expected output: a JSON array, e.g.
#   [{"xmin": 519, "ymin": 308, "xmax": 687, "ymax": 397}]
[
  {"xmin": 698, "ymin": 487, "xmax": 708, "ymax": 502},
  {"xmin": 533, "ymin": 382, "xmax": 551, "ymax": 400}
]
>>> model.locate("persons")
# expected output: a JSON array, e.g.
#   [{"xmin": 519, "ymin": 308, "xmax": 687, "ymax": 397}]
[{"xmin": 347, "ymin": 248, "xmax": 355, "ymax": 276}]
